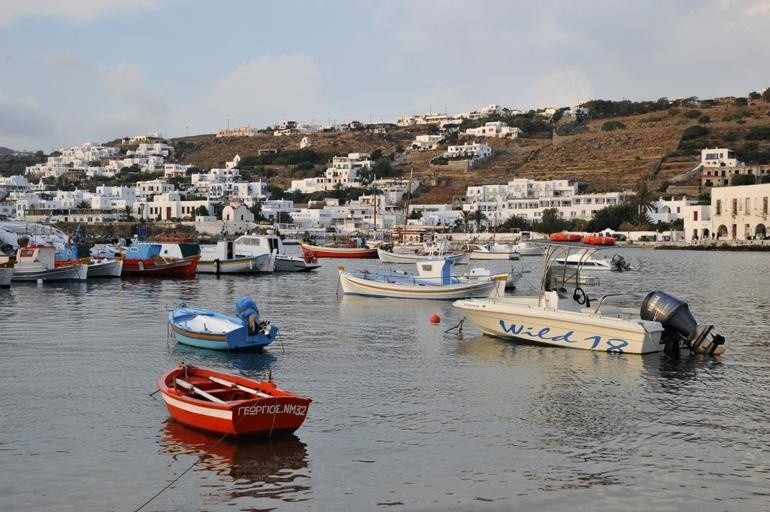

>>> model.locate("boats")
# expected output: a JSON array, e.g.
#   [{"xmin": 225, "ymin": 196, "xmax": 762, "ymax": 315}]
[
  {"xmin": 550, "ymin": 248, "xmax": 633, "ymax": 273},
  {"xmin": 157, "ymin": 361, "xmax": 313, "ymax": 440},
  {"xmin": 448, "ymin": 243, "xmax": 730, "ymax": 361},
  {"xmin": 336, "ymin": 254, "xmax": 536, "ymax": 301},
  {"xmin": 297, "ymin": 230, "xmax": 379, "ymax": 259},
  {"xmin": 375, "ymin": 228, "xmax": 617, "ymax": 267},
  {"xmin": 0, "ymin": 228, "xmax": 322, "ymax": 287}
]
